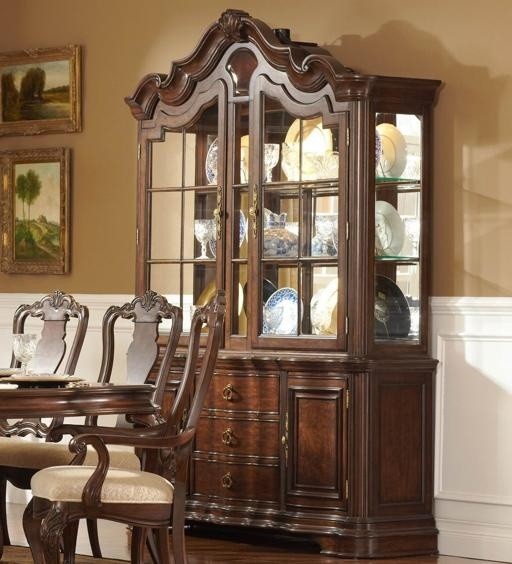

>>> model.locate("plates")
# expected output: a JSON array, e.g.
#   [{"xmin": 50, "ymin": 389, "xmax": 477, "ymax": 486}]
[
  {"xmin": 376, "ymin": 275, "xmax": 411, "ymax": 339},
  {"xmin": 196, "ymin": 278, "xmax": 304, "ymax": 335},
  {"xmin": 0, "ymin": 368, "xmax": 86, "ymax": 385},
  {"xmin": 205, "ymin": 133, "xmax": 254, "ymax": 185},
  {"xmin": 280, "ymin": 116, "xmax": 407, "ymax": 181},
  {"xmin": 208, "ymin": 205, "xmax": 246, "ymax": 258},
  {"xmin": 375, "ymin": 201, "xmax": 405, "ymax": 257}
]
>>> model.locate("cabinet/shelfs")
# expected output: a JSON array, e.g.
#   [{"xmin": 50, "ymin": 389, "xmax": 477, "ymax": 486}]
[{"xmin": 121, "ymin": 9, "xmax": 439, "ymax": 559}]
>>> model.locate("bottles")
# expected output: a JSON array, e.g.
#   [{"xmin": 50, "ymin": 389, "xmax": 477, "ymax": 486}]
[{"xmin": 259, "ymin": 211, "xmax": 338, "ymax": 257}]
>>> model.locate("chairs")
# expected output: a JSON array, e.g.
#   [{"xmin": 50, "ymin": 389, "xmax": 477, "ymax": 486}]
[
  {"xmin": 22, "ymin": 286, "xmax": 226, "ymax": 562},
  {"xmin": 1, "ymin": 289, "xmax": 183, "ymax": 554},
  {"xmin": 2, "ymin": 291, "xmax": 89, "ymax": 545}
]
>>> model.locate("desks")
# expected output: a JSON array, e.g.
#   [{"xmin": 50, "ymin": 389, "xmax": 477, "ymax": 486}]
[{"xmin": 3, "ymin": 371, "xmax": 163, "ymax": 564}]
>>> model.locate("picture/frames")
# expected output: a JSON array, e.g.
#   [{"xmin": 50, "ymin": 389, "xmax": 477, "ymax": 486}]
[{"xmin": 0, "ymin": 43, "xmax": 83, "ymax": 274}]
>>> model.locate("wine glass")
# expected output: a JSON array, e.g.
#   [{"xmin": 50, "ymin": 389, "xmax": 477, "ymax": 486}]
[
  {"xmin": 263, "ymin": 144, "xmax": 280, "ymax": 183},
  {"xmin": 194, "ymin": 219, "xmax": 211, "ymax": 261},
  {"xmin": 10, "ymin": 334, "xmax": 36, "ymax": 378}
]
